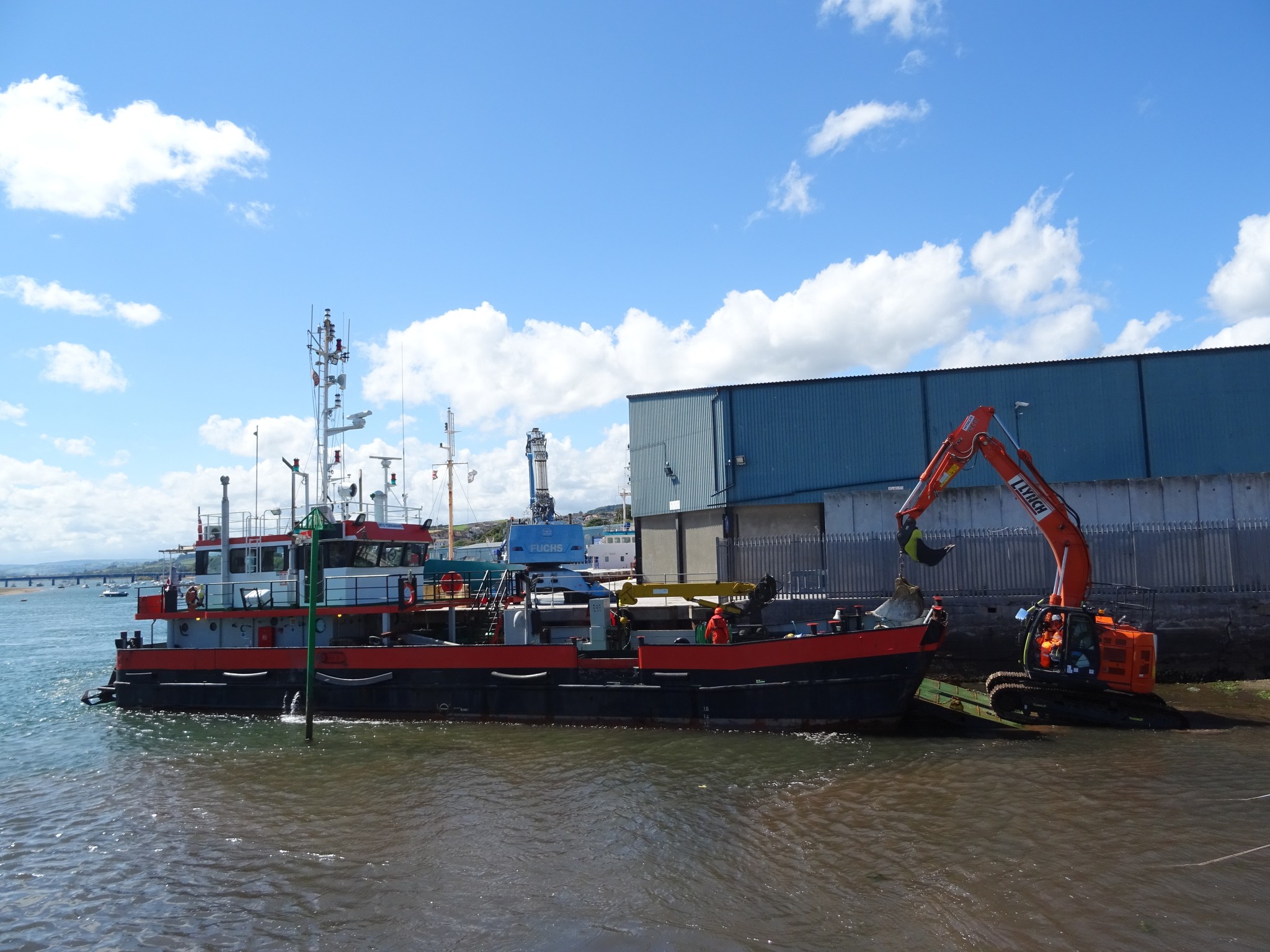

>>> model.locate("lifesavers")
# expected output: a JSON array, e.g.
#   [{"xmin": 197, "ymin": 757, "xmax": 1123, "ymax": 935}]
[
  {"xmin": 403, "ymin": 581, "xmax": 414, "ymax": 606},
  {"xmin": 185, "ymin": 585, "xmax": 203, "ymax": 609}
]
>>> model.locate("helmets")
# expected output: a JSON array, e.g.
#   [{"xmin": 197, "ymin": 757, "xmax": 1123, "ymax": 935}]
[
  {"xmin": 1051, "ymin": 614, "xmax": 1063, "ymax": 621},
  {"xmin": 714, "ymin": 607, "xmax": 723, "ymax": 615}
]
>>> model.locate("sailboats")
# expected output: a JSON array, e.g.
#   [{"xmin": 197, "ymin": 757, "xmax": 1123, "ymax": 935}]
[{"xmin": 57, "ymin": 560, "xmax": 195, "ymax": 588}]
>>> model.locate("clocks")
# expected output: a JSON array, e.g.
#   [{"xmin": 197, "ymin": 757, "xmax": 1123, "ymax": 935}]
[{"xmin": 316, "ymin": 619, "xmax": 327, "ymax": 634}]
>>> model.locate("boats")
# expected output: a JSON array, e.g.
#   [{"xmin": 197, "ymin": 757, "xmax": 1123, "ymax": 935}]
[
  {"xmin": 560, "ymin": 443, "xmax": 636, "ymax": 571},
  {"xmin": 80, "ymin": 304, "xmax": 950, "ymax": 729},
  {"xmin": 36, "ymin": 583, "xmax": 44, "ymax": 587},
  {"xmin": 102, "ymin": 585, "xmax": 129, "ymax": 597}
]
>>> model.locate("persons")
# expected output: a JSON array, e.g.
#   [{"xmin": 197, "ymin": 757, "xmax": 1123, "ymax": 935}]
[
  {"xmin": 188, "ymin": 581, "xmax": 195, "ymax": 610},
  {"xmin": 1037, "ymin": 614, "xmax": 1063, "ymax": 669},
  {"xmin": 705, "ymin": 607, "xmax": 730, "ymax": 644}
]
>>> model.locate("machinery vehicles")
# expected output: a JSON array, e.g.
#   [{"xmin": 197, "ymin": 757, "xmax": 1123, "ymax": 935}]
[{"xmin": 894, "ymin": 407, "xmax": 1168, "ymax": 727}]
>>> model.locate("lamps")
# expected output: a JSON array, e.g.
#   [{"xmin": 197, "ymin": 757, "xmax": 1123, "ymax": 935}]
[
  {"xmin": 1014, "ymin": 401, "xmax": 1028, "ymax": 410},
  {"xmin": 664, "ymin": 467, "xmax": 672, "ymax": 477},
  {"xmin": 352, "ymin": 513, "xmax": 366, "ymax": 526},
  {"xmin": 421, "ymin": 519, "xmax": 433, "ymax": 530},
  {"xmin": 337, "ymin": 482, "xmax": 358, "ymax": 498},
  {"xmin": 832, "ymin": 610, "xmax": 841, "ymax": 621},
  {"xmin": 735, "ymin": 455, "xmax": 746, "ymax": 465},
  {"xmin": 271, "ymin": 508, "xmax": 282, "ymax": 515}
]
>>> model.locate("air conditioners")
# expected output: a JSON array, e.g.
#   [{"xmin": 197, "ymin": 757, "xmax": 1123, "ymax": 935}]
[{"xmin": 204, "ymin": 525, "xmax": 222, "ymax": 540}]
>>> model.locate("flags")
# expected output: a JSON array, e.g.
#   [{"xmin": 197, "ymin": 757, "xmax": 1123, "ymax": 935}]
[
  {"xmin": 433, "ymin": 470, "xmax": 438, "ymax": 480},
  {"xmin": 164, "ymin": 577, "xmax": 172, "ymax": 590}
]
